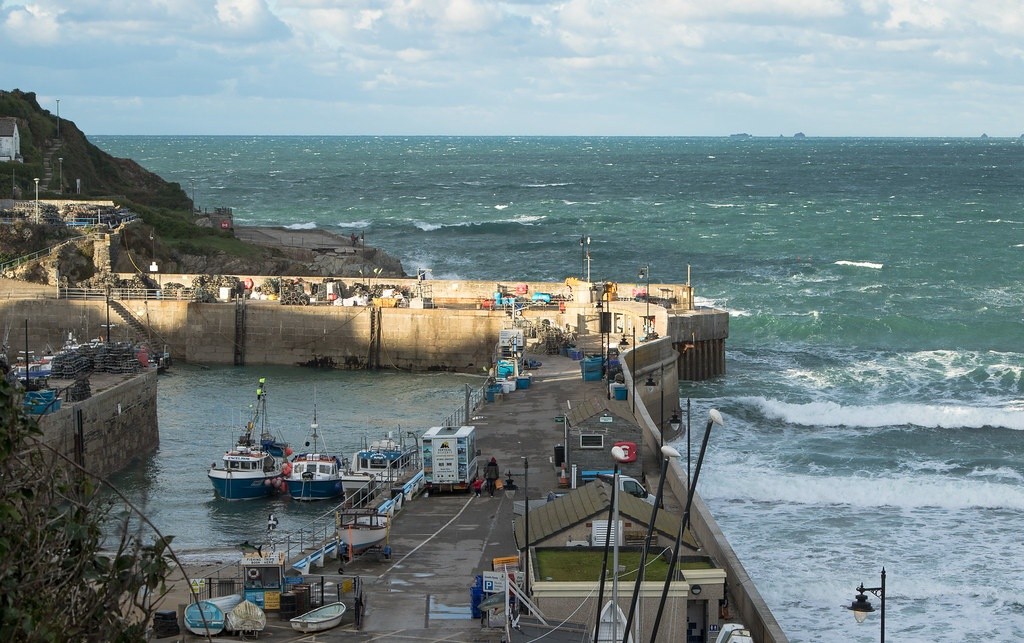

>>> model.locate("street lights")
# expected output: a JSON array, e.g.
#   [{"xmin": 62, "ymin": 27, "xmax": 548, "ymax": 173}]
[
  {"xmin": 579, "ymin": 235, "xmax": 585, "ymax": 281},
  {"xmin": 503, "ymin": 457, "xmax": 529, "ymax": 615},
  {"xmin": 34, "ymin": 177, "xmax": 41, "ymax": 225},
  {"xmin": 360, "ymin": 231, "xmax": 365, "ymax": 285},
  {"xmin": 150, "ymin": 227, "xmax": 156, "ymax": 280},
  {"xmin": 596, "ymin": 291, "xmax": 606, "ymax": 379},
  {"xmin": 58, "ymin": 157, "xmax": 63, "ymax": 194},
  {"xmin": 638, "ymin": 263, "xmax": 650, "ymax": 342},
  {"xmin": 847, "ymin": 566, "xmax": 886, "ymax": 643}
]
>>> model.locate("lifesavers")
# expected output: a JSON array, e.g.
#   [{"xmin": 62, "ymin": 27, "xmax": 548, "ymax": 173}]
[
  {"xmin": 296, "ymin": 456, "xmax": 306, "ymax": 461},
  {"xmin": 245, "ymin": 278, "xmax": 254, "ymax": 288},
  {"xmin": 322, "ymin": 457, "xmax": 332, "ymax": 461},
  {"xmin": 248, "ymin": 568, "xmax": 260, "ymax": 579}
]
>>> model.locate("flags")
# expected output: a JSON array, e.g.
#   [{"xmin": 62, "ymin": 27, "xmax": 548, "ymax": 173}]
[{"xmin": 268, "ymin": 514, "xmax": 278, "ymax": 531}]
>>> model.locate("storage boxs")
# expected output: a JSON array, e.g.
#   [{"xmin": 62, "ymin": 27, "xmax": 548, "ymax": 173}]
[
  {"xmin": 567, "ymin": 347, "xmax": 627, "ymax": 400},
  {"xmin": 24, "ymin": 390, "xmax": 62, "ymax": 414},
  {"xmin": 487, "ymin": 360, "xmax": 530, "ymax": 401},
  {"xmin": 496, "ymin": 293, "xmax": 516, "ymax": 305}
]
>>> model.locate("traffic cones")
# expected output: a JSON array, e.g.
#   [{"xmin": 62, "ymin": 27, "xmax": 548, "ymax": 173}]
[{"xmin": 558, "ymin": 463, "xmax": 569, "ymax": 487}]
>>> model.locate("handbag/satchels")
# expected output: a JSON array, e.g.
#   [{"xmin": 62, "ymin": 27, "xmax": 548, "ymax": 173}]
[{"xmin": 496, "ymin": 478, "xmax": 503, "ymax": 491}]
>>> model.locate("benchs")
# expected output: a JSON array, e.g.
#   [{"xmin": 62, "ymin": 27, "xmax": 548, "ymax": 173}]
[{"xmin": 581, "ymin": 470, "xmax": 621, "ymax": 484}]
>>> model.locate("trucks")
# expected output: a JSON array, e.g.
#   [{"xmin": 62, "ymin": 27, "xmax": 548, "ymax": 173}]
[{"xmin": 421, "ymin": 425, "xmax": 481, "ymax": 496}]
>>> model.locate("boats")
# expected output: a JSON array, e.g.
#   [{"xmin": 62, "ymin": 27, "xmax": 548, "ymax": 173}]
[
  {"xmin": 283, "ymin": 381, "xmax": 352, "ymax": 503},
  {"xmin": 339, "ymin": 416, "xmax": 420, "ymax": 503},
  {"xmin": 336, "ymin": 506, "xmax": 393, "ymax": 556},
  {"xmin": 207, "ymin": 374, "xmax": 293, "ymax": 504},
  {"xmin": 289, "ymin": 601, "xmax": 346, "ymax": 634}
]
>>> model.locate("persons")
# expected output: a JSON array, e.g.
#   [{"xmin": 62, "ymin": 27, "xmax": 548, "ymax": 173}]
[
  {"xmin": 484, "ymin": 457, "xmax": 499, "ymax": 498},
  {"xmin": 471, "ymin": 477, "xmax": 485, "ymax": 498}
]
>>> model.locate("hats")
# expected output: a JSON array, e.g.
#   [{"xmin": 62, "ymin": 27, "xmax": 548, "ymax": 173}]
[{"xmin": 491, "ymin": 456, "xmax": 496, "ymax": 462}]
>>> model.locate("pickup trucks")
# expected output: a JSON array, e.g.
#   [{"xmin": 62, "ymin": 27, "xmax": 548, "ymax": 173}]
[{"xmin": 513, "ymin": 474, "xmax": 656, "ymax": 516}]
